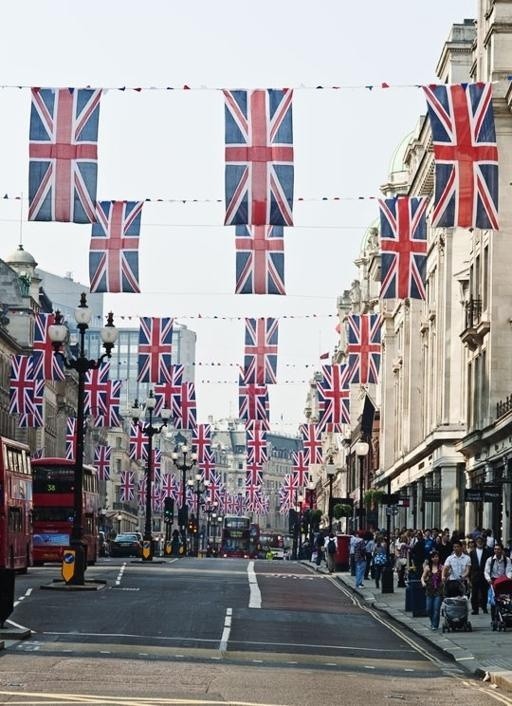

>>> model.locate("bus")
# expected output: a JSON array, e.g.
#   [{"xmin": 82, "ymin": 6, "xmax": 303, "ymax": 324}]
[
  {"xmin": 31, "ymin": 457, "xmax": 100, "ymax": 567},
  {"xmin": 220, "ymin": 515, "xmax": 251, "ymax": 559},
  {"xmin": 251, "ymin": 522, "xmax": 293, "ymax": 560},
  {"xmin": 0, "ymin": 436, "xmax": 35, "ymax": 574}
]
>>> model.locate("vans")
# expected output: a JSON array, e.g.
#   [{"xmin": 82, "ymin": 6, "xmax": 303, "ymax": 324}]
[{"xmin": 121, "ymin": 532, "xmax": 143, "ymax": 550}]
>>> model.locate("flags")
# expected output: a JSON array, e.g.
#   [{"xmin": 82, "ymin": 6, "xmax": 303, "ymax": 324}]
[
  {"xmin": 225, "ymin": 86, "xmax": 294, "ymax": 225},
  {"xmin": 234, "ymin": 224, "xmax": 285, "ymax": 295},
  {"xmin": 244, "ymin": 317, "xmax": 279, "ymax": 384},
  {"xmin": 137, "ymin": 318, "xmax": 172, "ymax": 383},
  {"xmin": 28, "ymin": 87, "xmax": 102, "ymax": 224},
  {"xmin": 10, "ymin": 356, "xmax": 351, "ymax": 516},
  {"xmin": 376, "ymin": 197, "xmax": 426, "ymax": 300},
  {"xmin": 89, "ymin": 201, "xmax": 143, "ymax": 293},
  {"xmin": 349, "ymin": 314, "xmax": 382, "ymax": 383},
  {"xmin": 422, "ymin": 84, "xmax": 498, "ymax": 227},
  {"xmin": 33, "ymin": 313, "xmax": 64, "ymax": 381}
]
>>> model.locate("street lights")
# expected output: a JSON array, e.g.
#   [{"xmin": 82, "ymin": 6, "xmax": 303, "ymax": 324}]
[
  {"xmin": 130, "ymin": 390, "xmax": 171, "ymax": 560},
  {"xmin": 48, "ymin": 290, "xmax": 118, "ymax": 586},
  {"xmin": 298, "ymin": 456, "xmax": 336, "ymax": 558},
  {"xmin": 355, "ymin": 436, "xmax": 369, "ymax": 530}
]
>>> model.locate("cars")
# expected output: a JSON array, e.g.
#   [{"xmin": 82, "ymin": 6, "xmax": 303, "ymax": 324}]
[{"xmin": 110, "ymin": 534, "xmax": 141, "ymax": 558}]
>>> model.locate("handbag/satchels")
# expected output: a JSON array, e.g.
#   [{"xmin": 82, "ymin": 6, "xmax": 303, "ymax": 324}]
[{"xmin": 399, "ymin": 556, "xmax": 407, "ymax": 566}]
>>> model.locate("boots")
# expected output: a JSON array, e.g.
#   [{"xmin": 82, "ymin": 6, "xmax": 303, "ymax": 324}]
[{"xmin": 396, "ymin": 568, "xmax": 407, "ymax": 589}]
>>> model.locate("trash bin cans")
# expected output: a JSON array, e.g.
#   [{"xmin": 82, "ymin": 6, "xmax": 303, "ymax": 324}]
[{"xmin": 410, "ymin": 580, "xmax": 427, "ymax": 617}]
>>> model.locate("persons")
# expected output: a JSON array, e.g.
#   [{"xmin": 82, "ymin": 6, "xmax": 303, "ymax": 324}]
[
  {"xmin": 205, "ymin": 544, "xmax": 212, "ymax": 558},
  {"xmin": 265, "ymin": 548, "xmax": 274, "ymax": 560},
  {"xmin": 300, "ymin": 521, "xmax": 512, "ymax": 631}
]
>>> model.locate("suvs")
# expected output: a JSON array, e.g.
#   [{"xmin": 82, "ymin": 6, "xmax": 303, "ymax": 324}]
[{"xmin": 99, "ymin": 531, "xmax": 108, "ymax": 557}]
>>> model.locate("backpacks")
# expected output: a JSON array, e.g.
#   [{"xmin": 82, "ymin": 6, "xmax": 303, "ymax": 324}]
[{"xmin": 327, "ymin": 538, "xmax": 336, "ymax": 554}]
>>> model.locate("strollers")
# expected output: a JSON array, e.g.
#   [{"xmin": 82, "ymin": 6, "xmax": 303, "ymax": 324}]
[{"xmin": 443, "ymin": 577, "xmax": 512, "ymax": 632}]
